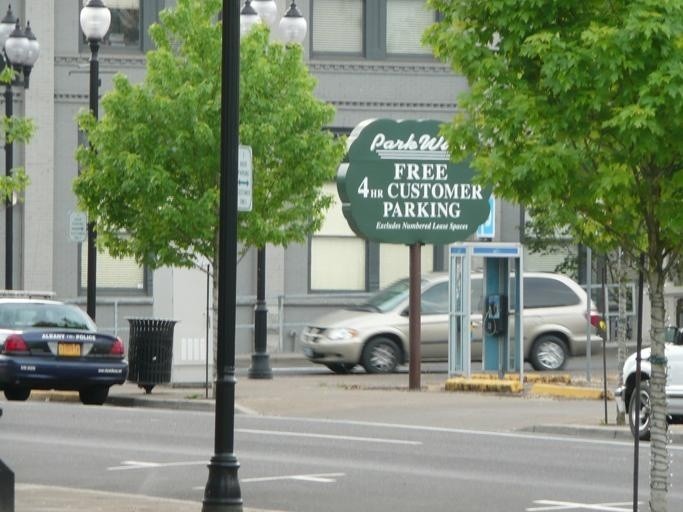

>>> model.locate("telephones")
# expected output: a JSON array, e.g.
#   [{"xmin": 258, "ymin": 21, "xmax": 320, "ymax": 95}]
[{"xmin": 488, "ymin": 295, "xmax": 502, "ymax": 320}]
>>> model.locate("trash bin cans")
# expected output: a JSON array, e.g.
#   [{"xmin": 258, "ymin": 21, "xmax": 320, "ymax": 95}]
[{"xmin": 127, "ymin": 317, "xmax": 177, "ymax": 394}]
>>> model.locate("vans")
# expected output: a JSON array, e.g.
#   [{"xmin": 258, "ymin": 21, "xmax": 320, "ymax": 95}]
[{"xmin": 299, "ymin": 270, "xmax": 604, "ymax": 373}]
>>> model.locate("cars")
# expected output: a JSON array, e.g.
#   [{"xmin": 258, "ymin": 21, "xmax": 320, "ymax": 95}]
[
  {"xmin": 615, "ymin": 296, "xmax": 683, "ymax": 440},
  {"xmin": 0, "ymin": 289, "xmax": 128, "ymax": 404}
]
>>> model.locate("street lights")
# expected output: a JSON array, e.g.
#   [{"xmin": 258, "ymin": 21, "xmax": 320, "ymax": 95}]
[
  {"xmin": 0, "ymin": 3, "xmax": 39, "ymax": 288},
  {"xmin": 240, "ymin": 1, "xmax": 306, "ymax": 377},
  {"xmin": 81, "ymin": 0, "xmax": 111, "ymax": 321}
]
